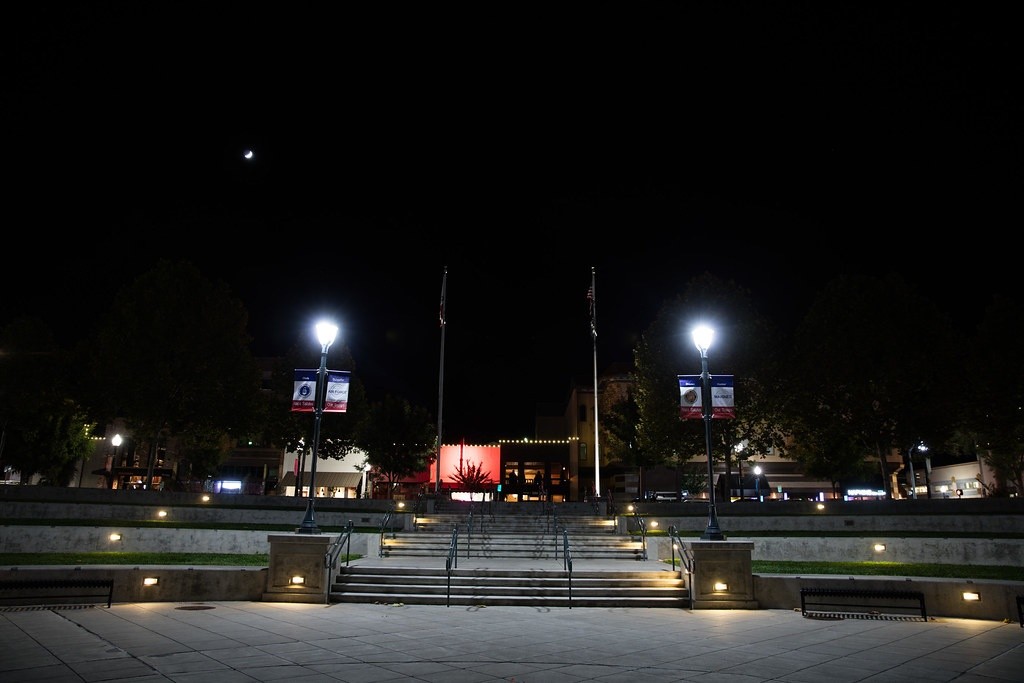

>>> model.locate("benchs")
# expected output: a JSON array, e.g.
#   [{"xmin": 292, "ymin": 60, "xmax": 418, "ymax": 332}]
[
  {"xmin": 0, "ymin": 578, "xmax": 115, "ymax": 608},
  {"xmin": 800, "ymin": 588, "xmax": 927, "ymax": 622}
]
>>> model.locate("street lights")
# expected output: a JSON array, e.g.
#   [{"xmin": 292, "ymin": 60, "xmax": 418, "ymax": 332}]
[
  {"xmin": 109, "ymin": 433, "xmax": 122, "ymax": 488},
  {"xmin": 918, "ymin": 439, "xmax": 932, "ymax": 499},
  {"xmin": 293, "ymin": 319, "xmax": 339, "ymax": 535},
  {"xmin": 691, "ymin": 324, "xmax": 727, "ymax": 540},
  {"xmin": 754, "ymin": 466, "xmax": 762, "ymax": 504}
]
